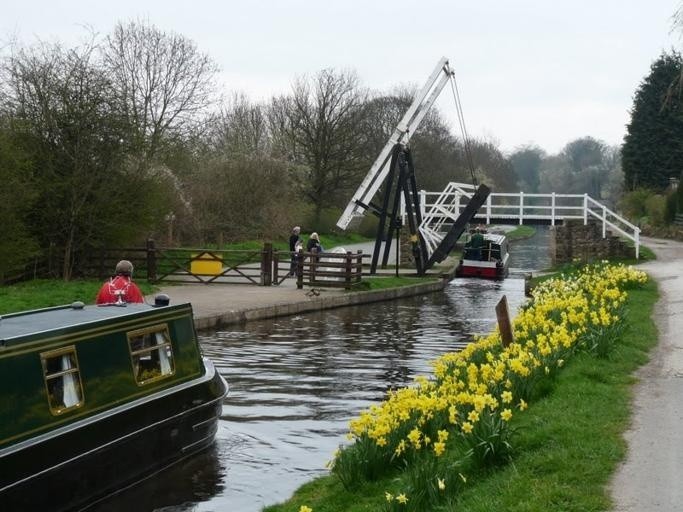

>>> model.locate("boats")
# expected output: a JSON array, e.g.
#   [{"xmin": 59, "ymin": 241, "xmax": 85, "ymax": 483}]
[
  {"xmin": 454, "ymin": 232, "xmax": 510, "ymax": 280},
  {"xmin": 0, "ymin": 293, "xmax": 229, "ymax": 512}
]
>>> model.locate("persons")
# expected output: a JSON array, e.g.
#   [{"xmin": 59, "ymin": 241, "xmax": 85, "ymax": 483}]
[
  {"xmin": 471, "ymin": 227, "xmax": 484, "ymax": 260},
  {"xmin": 307, "ymin": 232, "xmax": 322, "ymax": 268},
  {"xmin": 96, "ymin": 260, "xmax": 145, "ymax": 377},
  {"xmin": 290, "ymin": 226, "xmax": 301, "ymax": 276}
]
